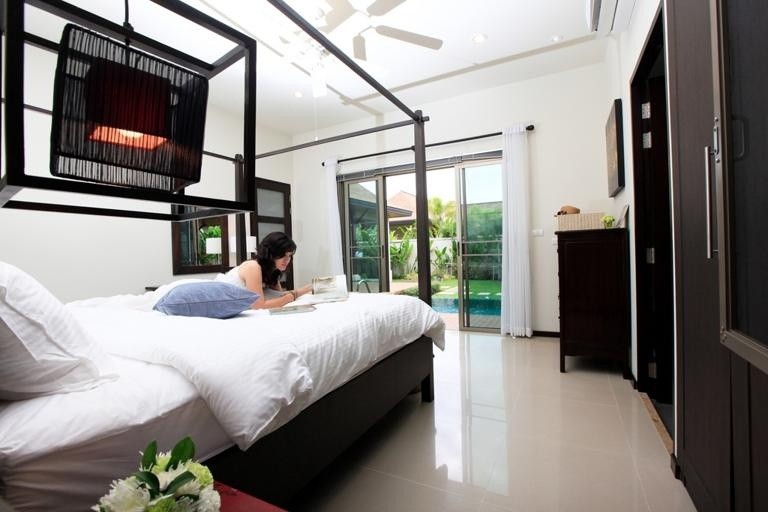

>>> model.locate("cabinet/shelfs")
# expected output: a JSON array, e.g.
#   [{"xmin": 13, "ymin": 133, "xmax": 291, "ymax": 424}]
[
  {"xmin": 554, "ymin": 227, "xmax": 631, "ymax": 379},
  {"xmin": 665, "ymin": 0, "xmax": 768, "ymax": 512}
]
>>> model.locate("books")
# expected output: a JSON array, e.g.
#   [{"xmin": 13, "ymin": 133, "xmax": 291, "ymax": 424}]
[{"xmin": 282, "ymin": 274, "xmax": 350, "ymax": 309}]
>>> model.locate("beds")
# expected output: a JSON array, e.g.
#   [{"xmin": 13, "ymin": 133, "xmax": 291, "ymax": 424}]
[{"xmin": 0, "ymin": 290, "xmax": 445, "ymax": 504}]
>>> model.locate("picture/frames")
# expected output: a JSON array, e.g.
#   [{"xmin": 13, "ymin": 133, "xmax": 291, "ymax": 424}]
[{"xmin": 605, "ymin": 98, "xmax": 625, "ymax": 198}]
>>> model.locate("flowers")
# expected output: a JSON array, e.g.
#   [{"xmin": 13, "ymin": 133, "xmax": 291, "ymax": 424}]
[{"xmin": 89, "ymin": 431, "xmax": 221, "ymax": 512}]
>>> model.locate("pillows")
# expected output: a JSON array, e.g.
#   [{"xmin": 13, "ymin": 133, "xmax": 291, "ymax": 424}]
[
  {"xmin": 0, "ymin": 261, "xmax": 117, "ymax": 402},
  {"xmin": 152, "ymin": 281, "xmax": 261, "ymax": 319}
]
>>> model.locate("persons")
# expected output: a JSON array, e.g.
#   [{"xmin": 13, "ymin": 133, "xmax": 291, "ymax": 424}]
[{"xmin": 65, "ymin": 231, "xmax": 314, "ymax": 310}]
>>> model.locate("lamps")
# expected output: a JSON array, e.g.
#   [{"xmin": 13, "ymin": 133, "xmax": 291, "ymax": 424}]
[{"xmin": 49, "ymin": 0, "xmax": 209, "ymax": 192}]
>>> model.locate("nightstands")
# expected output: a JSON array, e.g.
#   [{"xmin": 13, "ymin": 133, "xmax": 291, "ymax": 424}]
[{"xmin": 213, "ymin": 479, "xmax": 288, "ymax": 512}]
